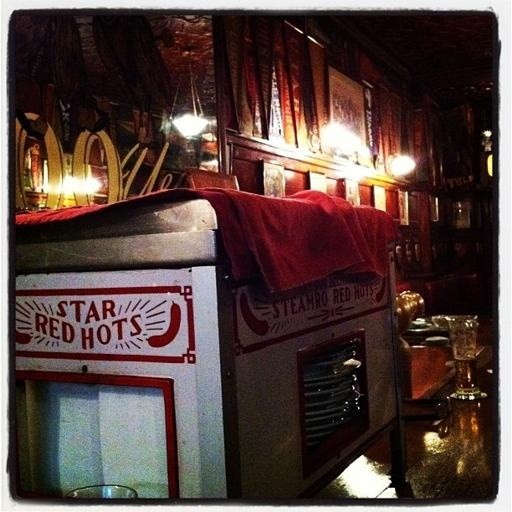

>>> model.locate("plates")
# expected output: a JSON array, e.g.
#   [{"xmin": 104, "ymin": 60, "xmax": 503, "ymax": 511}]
[{"xmin": 299, "ymin": 334, "xmax": 363, "ymax": 450}]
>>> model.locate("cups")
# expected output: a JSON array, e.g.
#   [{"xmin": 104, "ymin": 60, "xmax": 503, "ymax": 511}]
[
  {"xmin": 65, "ymin": 484, "xmax": 140, "ymax": 498},
  {"xmin": 445, "ymin": 313, "xmax": 490, "ymax": 402}
]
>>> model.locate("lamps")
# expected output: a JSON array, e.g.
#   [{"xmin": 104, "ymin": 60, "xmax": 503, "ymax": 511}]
[{"xmin": 168, "ymin": 66, "xmax": 210, "ymax": 140}]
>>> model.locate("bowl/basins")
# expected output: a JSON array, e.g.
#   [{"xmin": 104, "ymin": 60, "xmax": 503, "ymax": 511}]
[{"xmin": 426, "ymin": 336, "xmax": 449, "ymax": 346}]
